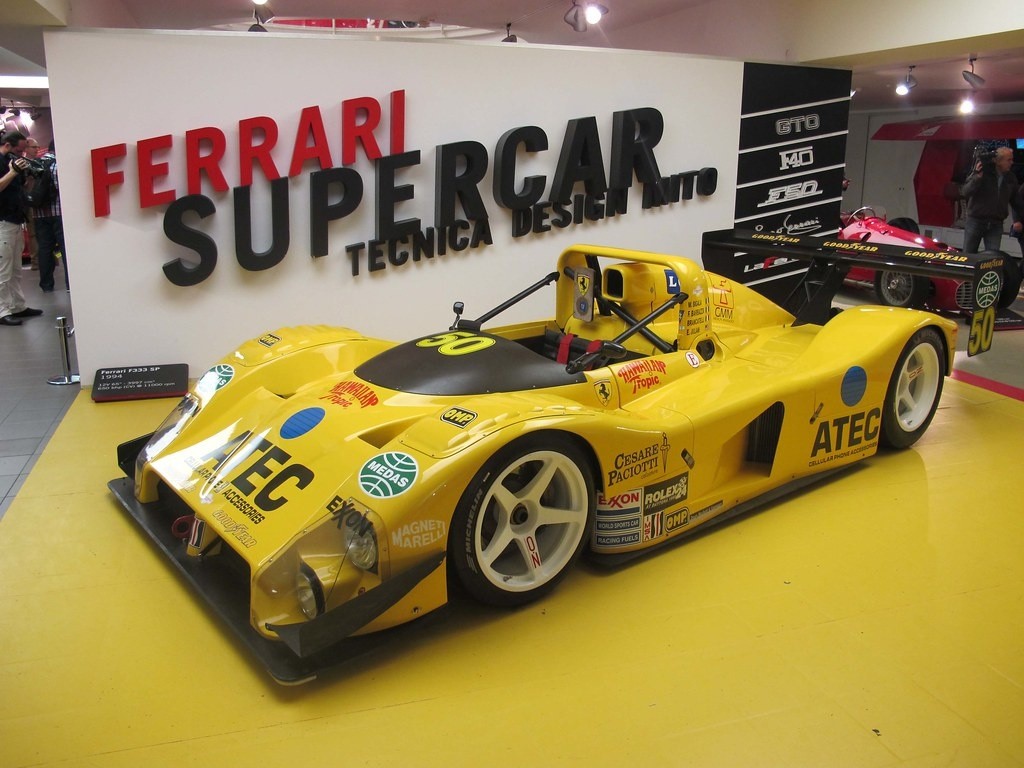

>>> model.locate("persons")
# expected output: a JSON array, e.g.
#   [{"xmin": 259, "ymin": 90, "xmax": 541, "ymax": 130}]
[
  {"xmin": 0, "ymin": 130, "xmax": 69, "ymax": 326},
  {"xmin": 842, "ymin": 179, "xmax": 850, "ymax": 192},
  {"xmin": 962, "ymin": 147, "xmax": 1024, "ymax": 293}
]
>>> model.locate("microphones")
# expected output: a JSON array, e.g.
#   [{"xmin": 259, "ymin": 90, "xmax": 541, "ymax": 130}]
[{"xmin": 21, "ymin": 156, "xmax": 42, "ymax": 168}]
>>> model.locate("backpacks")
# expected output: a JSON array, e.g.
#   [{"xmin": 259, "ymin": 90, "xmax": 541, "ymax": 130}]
[{"xmin": 17, "ymin": 156, "xmax": 57, "ymax": 209}]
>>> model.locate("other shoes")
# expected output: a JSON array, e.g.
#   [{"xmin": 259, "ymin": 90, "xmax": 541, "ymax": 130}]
[
  {"xmin": 42, "ymin": 287, "xmax": 53, "ymax": 292},
  {"xmin": 31, "ymin": 264, "xmax": 39, "ymax": 270},
  {"xmin": 55, "ymin": 256, "xmax": 59, "ymax": 265},
  {"xmin": 66, "ymin": 284, "xmax": 70, "ymax": 293}
]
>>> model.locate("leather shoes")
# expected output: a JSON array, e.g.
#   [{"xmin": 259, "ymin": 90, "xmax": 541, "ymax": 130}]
[
  {"xmin": 0, "ymin": 315, "xmax": 22, "ymax": 325},
  {"xmin": 13, "ymin": 307, "xmax": 43, "ymax": 317}
]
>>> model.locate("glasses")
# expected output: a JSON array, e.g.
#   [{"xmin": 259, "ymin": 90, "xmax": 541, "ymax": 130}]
[{"xmin": 27, "ymin": 146, "xmax": 41, "ymax": 149}]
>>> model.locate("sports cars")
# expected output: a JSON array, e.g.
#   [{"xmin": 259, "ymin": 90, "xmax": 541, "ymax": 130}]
[
  {"xmin": 836, "ymin": 205, "xmax": 1021, "ymax": 315},
  {"xmin": 104, "ymin": 241, "xmax": 961, "ymax": 690}
]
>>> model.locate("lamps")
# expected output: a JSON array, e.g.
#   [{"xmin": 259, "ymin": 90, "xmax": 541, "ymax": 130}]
[
  {"xmin": 254, "ymin": 5, "xmax": 275, "ymax": 24},
  {"xmin": 31, "ymin": 107, "xmax": 41, "ymax": 121},
  {"xmin": 962, "ymin": 56, "xmax": 985, "ymax": 89},
  {"xmin": 502, "ymin": 28, "xmax": 516, "ymax": 42},
  {"xmin": 9, "ymin": 100, "xmax": 21, "ymax": 116},
  {"xmin": 896, "ymin": 66, "xmax": 917, "ymax": 96},
  {"xmin": 565, "ymin": 0, "xmax": 608, "ymax": 32}
]
